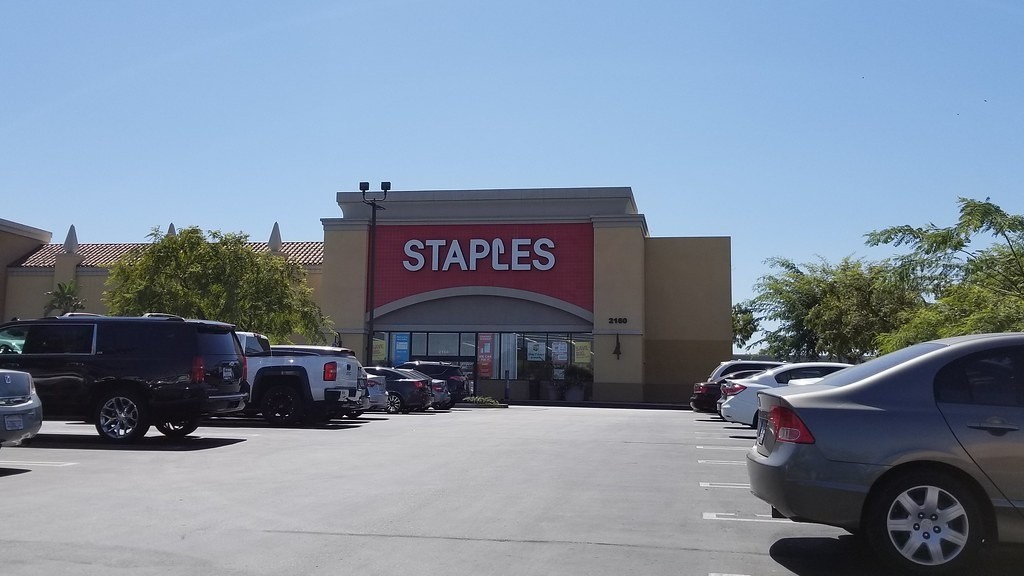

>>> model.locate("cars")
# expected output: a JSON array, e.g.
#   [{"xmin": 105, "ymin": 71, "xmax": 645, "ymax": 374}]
[
  {"xmin": 363, "ymin": 365, "xmax": 433, "ymax": 413},
  {"xmin": 689, "ymin": 370, "xmax": 769, "ymax": 416},
  {"xmin": 345, "ymin": 368, "xmax": 388, "ymax": 418},
  {"xmin": 0, "ymin": 368, "xmax": 43, "ymax": 450},
  {"xmin": 747, "ymin": 332, "xmax": 1024, "ymax": 576},
  {"xmin": 393, "ymin": 368, "xmax": 450, "ymax": 409},
  {"xmin": 719, "ymin": 361, "xmax": 857, "ymax": 428}
]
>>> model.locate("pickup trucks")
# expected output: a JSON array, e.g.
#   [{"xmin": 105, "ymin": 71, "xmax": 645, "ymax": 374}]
[{"xmin": 203, "ymin": 332, "xmax": 359, "ymax": 427}]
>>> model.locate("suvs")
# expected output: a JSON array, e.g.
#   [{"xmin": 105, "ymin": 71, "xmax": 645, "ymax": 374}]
[{"xmin": 394, "ymin": 361, "xmax": 469, "ymax": 410}]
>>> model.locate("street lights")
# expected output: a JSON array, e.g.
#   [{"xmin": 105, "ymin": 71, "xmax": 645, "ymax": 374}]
[{"xmin": 359, "ymin": 182, "xmax": 391, "ymax": 367}]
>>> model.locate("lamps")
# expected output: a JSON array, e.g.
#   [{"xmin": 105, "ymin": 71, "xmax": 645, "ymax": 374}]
[
  {"xmin": 332, "ymin": 332, "xmax": 343, "ymax": 347},
  {"xmin": 612, "ymin": 333, "xmax": 622, "ymax": 360}
]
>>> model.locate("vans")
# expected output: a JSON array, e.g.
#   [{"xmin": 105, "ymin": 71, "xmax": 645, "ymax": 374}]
[
  {"xmin": 0, "ymin": 310, "xmax": 249, "ymax": 444},
  {"xmin": 270, "ymin": 345, "xmax": 363, "ymax": 417},
  {"xmin": 707, "ymin": 358, "xmax": 788, "ymax": 383}
]
